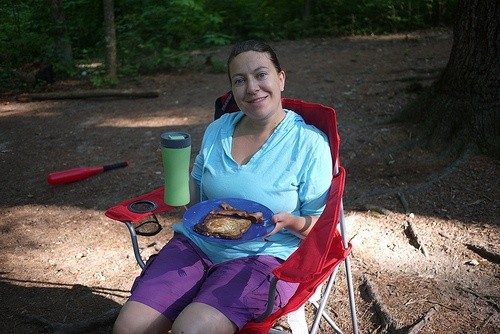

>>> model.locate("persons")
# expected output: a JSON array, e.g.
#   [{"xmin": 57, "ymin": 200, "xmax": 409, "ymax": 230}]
[{"xmin": 111, "ymin": 41, "xmax": 333, "ymax": 334}]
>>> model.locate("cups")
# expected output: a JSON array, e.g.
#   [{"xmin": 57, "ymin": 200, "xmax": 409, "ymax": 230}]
[{"xmin": 161, "ymin": 132, "xmax": 191, "ymax": 207}]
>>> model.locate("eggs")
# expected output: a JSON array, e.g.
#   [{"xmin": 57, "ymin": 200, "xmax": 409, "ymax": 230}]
[{"xmin": 207, "ymin": 219, "xmax": 233, "ymax": 232}]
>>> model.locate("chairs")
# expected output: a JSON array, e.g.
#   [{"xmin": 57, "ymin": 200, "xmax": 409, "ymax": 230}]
[{"xmin": 104, "ymin": 91, "xmax": 358, "ymax": 334}]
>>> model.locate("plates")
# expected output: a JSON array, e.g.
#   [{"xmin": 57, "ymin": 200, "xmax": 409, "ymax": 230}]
[{"xmin": 183, "ymin": 198, "xmax": 276, "ymax": 244}]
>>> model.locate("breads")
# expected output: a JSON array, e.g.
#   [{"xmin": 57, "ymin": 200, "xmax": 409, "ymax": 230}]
[{"xmin": 194, "ymin": 213, "xmax": 252, "ymax": 239}]
